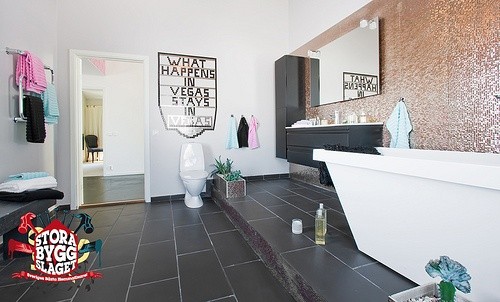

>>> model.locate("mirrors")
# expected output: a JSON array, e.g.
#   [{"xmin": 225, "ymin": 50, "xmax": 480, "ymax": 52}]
[{"xmin": 310, "ymin": 16, "xmax": 380, "ymax": 106}]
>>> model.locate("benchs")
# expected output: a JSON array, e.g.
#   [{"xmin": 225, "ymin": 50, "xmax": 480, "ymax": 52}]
[{"xmin": 0, "ymin": 186, "xmax": 56, "ymax": 252}]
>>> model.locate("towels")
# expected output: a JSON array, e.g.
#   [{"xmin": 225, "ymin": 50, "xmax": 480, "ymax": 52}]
[
  {"xmin": 9, "ymin": 171, "xmax": 48, "ymax": 180},
  {"xmin": 1, "ymin": 189, "xmax": 64, "ymax": 202},
  {"xmin": 43, "ymin": 82, "xmax": 60, "ymax": 125},
  {"xmin": 237, "ymin": 117, "xmax": 249, "ymax": 148},
  {"xmin": 0, "ymin": 175, "xmax": 57, "ymax": 192},
  {"xmin": 248, "ymin": 117, "xmax": 259, "ymax": 149},
  {"xmin": 225, "ymin": 117, "xmax": 240, "ymax": 148},
  {"xmin": 385, "ymin": 100, "xmax": 413, "ymax": 148},
  {"xmin": 16, "ymin": 51, "xmax": 48, "ymax": 97},
  {"xmin": 24, "ymin": 93, "xmax": 46, "ymax": 143}
]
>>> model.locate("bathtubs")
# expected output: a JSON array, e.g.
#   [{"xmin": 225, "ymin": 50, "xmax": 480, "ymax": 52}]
[{"xmin": 311, "ymin": 146, "xmax": 499, "ymax": 302}]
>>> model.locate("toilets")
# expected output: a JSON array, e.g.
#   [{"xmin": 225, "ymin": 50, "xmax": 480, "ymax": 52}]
[{"xmin": 179, "ymin": 141, "xmax": 207, "ymax": 209}]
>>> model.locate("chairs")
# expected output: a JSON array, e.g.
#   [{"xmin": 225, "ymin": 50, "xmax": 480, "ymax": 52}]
[{"xmin": 85, "ymin": 135, "xmax": 103, "ymax": 163}]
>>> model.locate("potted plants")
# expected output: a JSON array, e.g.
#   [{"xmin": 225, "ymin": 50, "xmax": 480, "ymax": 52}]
[{"xmin": 211, "ymin": 157, "xmax": 246, "ymax": 200}]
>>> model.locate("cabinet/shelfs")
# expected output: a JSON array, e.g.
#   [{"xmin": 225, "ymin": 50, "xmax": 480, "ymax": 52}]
[
  {"xmin": 273, "ymin": 55, "xmax": 305, "ymax": 159},
  {"xmin": 309, "ymin": 56, "xmax": 321, "ymax": 106},
  {"xmin": 285, "ymin": 124, "xmax": 383, "ymax": 184}
]
